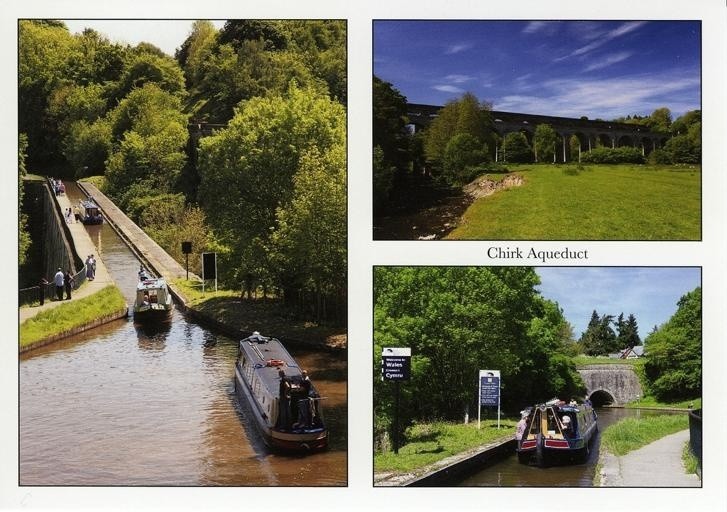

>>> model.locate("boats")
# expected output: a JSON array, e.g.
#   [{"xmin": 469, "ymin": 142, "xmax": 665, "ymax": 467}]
[
  {"xmin": 131, "ymin": 277, "xmax": 174, "ymax": 327},
  {"xmin": 76, "ymin": 199, "xmax": 104, "ymax": 225},
  {"xmin": 515, "ymin": 398, "xmax": 603, "ymax": 469},
  {"xmin": 233, "ymin": 331, "xmax": 330, "ymax": 457}
]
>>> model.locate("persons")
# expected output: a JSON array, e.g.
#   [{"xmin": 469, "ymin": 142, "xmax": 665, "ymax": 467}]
[
  {"xmin": 65, "ymin": 205, "xmax": 82, "ymax": 223},
  {"xmin": 583, "ymin": 395, "xmax": 592, "ymax": 409},
  {"xmin": 64, "ymin": 270, "xmax": 73, "ymax": 300},
  {"xmin": 85, "ymin": 256, "xmax": 94, "ymax": 280},
  {"xmin": 52, "ymin": 178, "xmax": 65, "ymax": 196},
  {"xmin": 569, "ymin": 398, "xmax": 577, "ymax": 404},
  {"xmin": 137, "ymin": 265, "xmax": 152, "ymax": 281},
  {"xmin": 280, "ymin": 369, "xmax": 316, "ymax": 424},
  {"xmin": 91, "ymin": 254, "xmax": 96, "ymax": 278},
  {"xmin": 55, "ymin": 268, "xmax": 65, "ymax": 301}
]
